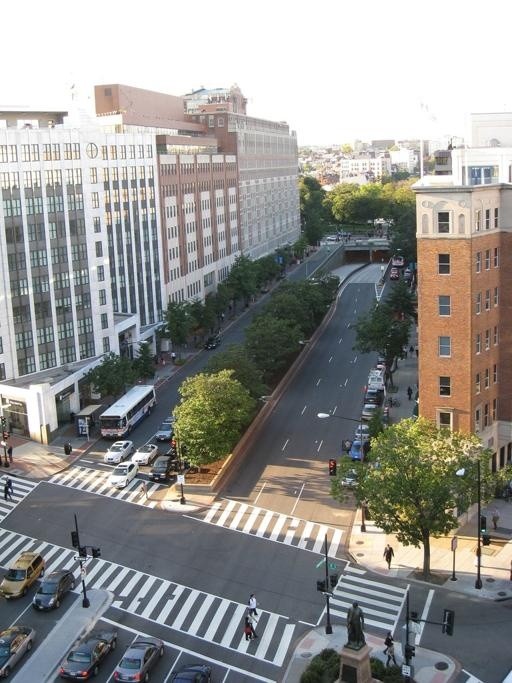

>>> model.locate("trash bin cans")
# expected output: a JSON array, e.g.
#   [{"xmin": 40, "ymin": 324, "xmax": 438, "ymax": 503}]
[{"xmin": 64, "ymin": 443, "xmax": 72, "ymax": 456}]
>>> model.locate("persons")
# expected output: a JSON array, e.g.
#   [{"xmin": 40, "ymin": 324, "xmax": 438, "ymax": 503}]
[
  {"xmin": 243, "ymin": 616, "xmax": 254, "ymax": 640},
  {"xmin": 409, "ymin": 345, "xmax": 414, "ymax": 358},
  {"xmin": 490, "ymin": 505, "xmax": 503, "ymax": 530},
  {"xmin": 248, "ymin": 593, "xmax": 257, "ymax": 615},
  {"xmin": 345, "ymin": 599, "xmax": 365, "ymax": 646},
  {"xmin": 7, "ymin": 445, "xmax": 14, "ymax": 463},
  {"xmin": 247, "ymin": 611, "xmax": 259, "ymax": 637},
  {"xmin": 6, "ymin": 477, "xmax": 14, "ymax": 493},
  {"xmin": 140, "ymin": 480, "xmax": 150, "ymax": 501},
  {"xmin": 383, "ymin": 543, "xmax": 394, "ymax": 570},
  {"xmin": 385, "ymin": 642, "xmax": 398, "ymax": 667},
  {"xmin": 407, "ymin": 385, "xmax": 413, "ymax": 400},
  {"xmin": 383, "ymin": 630, "xmax": 395, "ymax": 654},
  {"xmin": 3, "ymin": 483, "xmax": 13, "ymax": 500},
  {"xmin": 404, "ymin": 346, "xmax": 408, "ymax": 357}
]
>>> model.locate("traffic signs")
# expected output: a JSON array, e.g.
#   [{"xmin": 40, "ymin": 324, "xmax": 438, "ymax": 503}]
[{"xmin": 400, "ymin": 664, "xmax": 413, "ymax": 677}]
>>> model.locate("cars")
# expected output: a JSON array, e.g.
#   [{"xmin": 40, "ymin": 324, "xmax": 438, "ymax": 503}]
[
  {"xmin": 104, "ymin": 416, "xmax": 176, "ymax": 489},
  {"xmin": 0, "ymin": 625, "xmax": 36, "ymax": 680},
  {"xmin": 390, "ymin": 267, "xmax": 411, "ymax": 280},
  {"xmin": 0, "ymin": 551, "xmax": 75, "ymax": 612},
  {"xmin": 326, "ymin": 235, "xmax": 342, "ymax": 241},
  {"xmin": 341, "ymin": 355, "xmax": 386, "ymax": 490},
  {"xmin": 204, "ymin": 335, "xmax": 220, "ymax": 349},
  {"xmin": 60, "ymin": 629, "xmax": 212, "ymax": 683}
]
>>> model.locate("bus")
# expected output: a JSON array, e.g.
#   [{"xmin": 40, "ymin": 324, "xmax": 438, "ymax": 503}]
[
  {"xmin": 391, "ymin": 255, "xmax": 404, "ymax": 267},
  {"xmin": 99, "ymin": 384, "xmax": 156, "ymax": 438}
]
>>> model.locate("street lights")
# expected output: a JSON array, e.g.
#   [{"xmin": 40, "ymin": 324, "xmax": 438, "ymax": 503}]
[
  {"xmin": 85, "ymin": 417, "xmax": 90, "ymax": 442},
  {"xmin": 221, "ymin": 311, "xmax": 225, "ymax": 345},
  {"xmin": 119, "ymin": 340, "xmax": 148, "ymax": 347},
  {"xmin": 455, "ymin": 460, "xmax": 483, "ymax": 590}
]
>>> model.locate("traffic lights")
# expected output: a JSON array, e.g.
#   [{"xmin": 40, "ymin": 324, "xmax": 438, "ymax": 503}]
[
  {"xmin": 329, "ymin": 459, "xmax": 337, "ymax": 476},
  {"xmin": 71, "ymin": 531, "xmax": 101, "ymax": 559},
  {"xmin": 316, "ymin": 573, "xmax": 338, "ymax": 593},
  {"xmin": 480, "ymin": 516, "xmax": 491, "ymax": 546},
  {"xmin": 2, "ymin": 417, "xmax": 10, "ymax": 439},
  {"xmin": 405, "ymin": 645, "xmax": 415, "ymax": 659}
]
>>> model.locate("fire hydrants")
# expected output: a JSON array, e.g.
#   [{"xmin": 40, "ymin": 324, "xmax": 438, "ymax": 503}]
[
  {"xmin": 64, "ymin": 442, "xmax": 72, "ymax": 455},
  {"xmin": 383, "ymin": 405, "xmax": 389, "ymax": 417}
]
